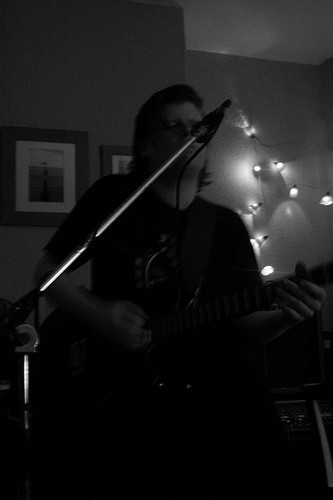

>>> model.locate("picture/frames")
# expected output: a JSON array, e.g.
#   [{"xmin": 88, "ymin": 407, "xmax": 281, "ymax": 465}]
[
  {"xmin": 99, "ymin": 145, "xmax": 135, "ymax": 177},
  {"xmin": 0, "ymin": 126, "xmax": 90, "ymax": 227}
]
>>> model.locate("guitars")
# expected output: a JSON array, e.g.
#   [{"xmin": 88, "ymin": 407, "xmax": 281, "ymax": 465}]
[{"xmin": 32, "ymin": 260, "xmax": 332, "ymax": 417}]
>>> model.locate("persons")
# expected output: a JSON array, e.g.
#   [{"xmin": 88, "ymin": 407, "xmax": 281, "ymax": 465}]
[{"xmin": 32, "ymin": 85, "xmax": 327, "ymax": 500}]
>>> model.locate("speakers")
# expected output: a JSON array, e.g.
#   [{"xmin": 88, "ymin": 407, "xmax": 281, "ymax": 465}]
[{"xmin": 260, "ymin": 309, "xmax": 327, "ymax": 398}]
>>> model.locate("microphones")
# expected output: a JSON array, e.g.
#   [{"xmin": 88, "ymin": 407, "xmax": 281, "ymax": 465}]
[{"xmin": 190, "ymin": 99, "xmax": 231, "ymax": 143}]
